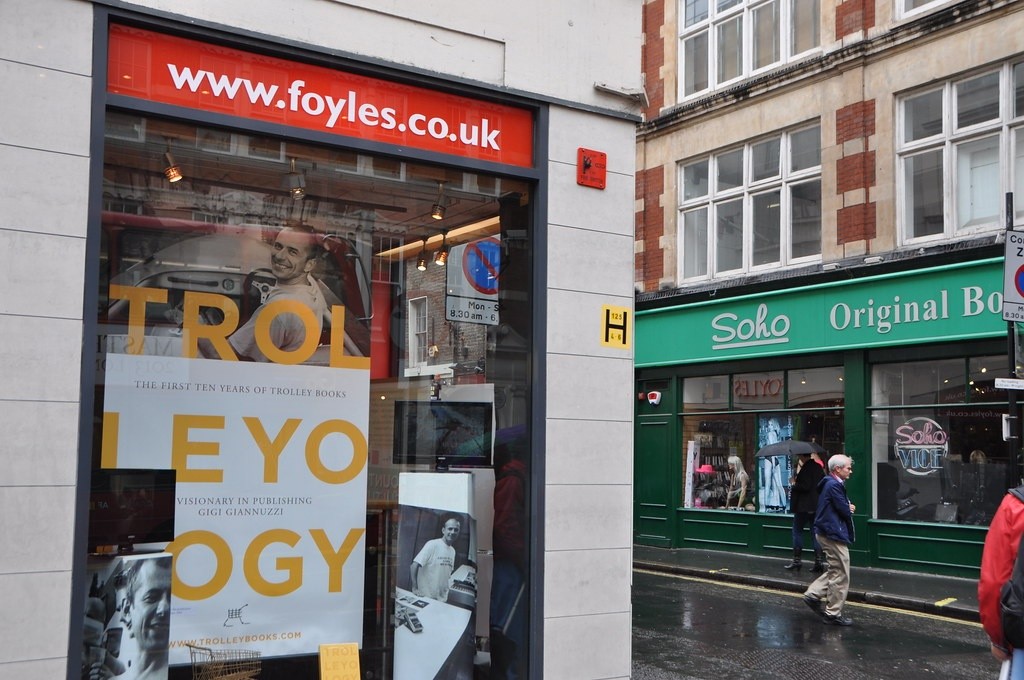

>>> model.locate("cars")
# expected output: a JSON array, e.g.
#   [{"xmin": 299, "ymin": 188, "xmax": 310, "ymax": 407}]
[{"xmin": 695, "ymin": 483, "xmax": 748, "ymax": 508}]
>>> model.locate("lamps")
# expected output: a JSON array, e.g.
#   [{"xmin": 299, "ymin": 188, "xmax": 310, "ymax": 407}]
[
  {"xmin": 417, "ymin": 235, "xmax": 430, "ymax": 271},
  {"xmin": 287, "ymin": 158, "xmax": 306, "ymax": 201},
  {"xmin": 435, "ymin": 229, "xmax": 451, "ymax": 265},
  {"xmin": 160, "ymin": 137, "xmax": 184, "ymax": 183},
  {"xmin": 430, "ymin": 180, "xmax": 448, "ymax": 220}
]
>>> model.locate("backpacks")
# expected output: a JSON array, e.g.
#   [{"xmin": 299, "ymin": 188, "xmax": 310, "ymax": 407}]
[{"xmin": 1000, "ymin": 485, "xmax": 1024, "ymax": 648}]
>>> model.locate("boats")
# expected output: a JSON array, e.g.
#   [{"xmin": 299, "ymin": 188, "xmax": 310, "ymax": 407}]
[{"xmin": 103, "ymin": 228, "xmax": 372, "ymax": 369}]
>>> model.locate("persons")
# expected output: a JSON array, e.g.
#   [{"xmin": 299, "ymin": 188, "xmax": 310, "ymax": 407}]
[
  {"xmin": 174, "ymin": 226, "xmax": 328, "ymax": 363},
  {"xmin": 82, "ymin": 556, "xmax": 172, "ymax": 680},
  {"xmin": 409, "ymin": 516, "xmax": 461, "ymax": 603},
  {"xmin": 977, "ymin": 477, "xmax": 1024, "ymax": 679},
  {"xmin": 784, "ymin": 453, "xmax": 825, "ymax": 572},
  {"xmin": 796, "ymin": 454, "xmax": 824, "ymax": 475},
  {"xmin": 804, "ymin": 454, "xmax": 856, "ymax": 625},
  {"xmin": 489, "ymin": 438, "xmax": 528, "ymax": 679},
  {"xmin": 725, "ymin": 456, "xmax": 755, "ymax": 511},
  {"xmin": 763, "ymin": 417, "xmax": 788, "ymax": 513}
]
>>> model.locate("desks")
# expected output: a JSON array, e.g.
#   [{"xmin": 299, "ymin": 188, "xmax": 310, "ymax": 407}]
[
  {"xmin": 393, "ymin": 586, "xmax": 472, "ymax": 680},
  {"xmin": 448, "ymin": 563, "xmax": 475, "ymax": 608}
]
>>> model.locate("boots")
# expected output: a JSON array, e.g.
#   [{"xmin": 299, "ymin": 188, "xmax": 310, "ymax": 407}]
[
  {"xmin": 808, "ymin": 550, "xmax": 824, "ymax": 573},
  {"xmin": 784, "ymin": 547, "xmax": 802, "ymax": 572}
]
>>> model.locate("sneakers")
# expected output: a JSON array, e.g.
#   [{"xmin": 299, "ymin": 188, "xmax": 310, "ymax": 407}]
[
  {"xmin": 823, "ymin": 612, "xmax": 852, "ymax": 625},
  {"xmin": 803, "ymin": 596, "xmax": 825, "ymax": 617}
]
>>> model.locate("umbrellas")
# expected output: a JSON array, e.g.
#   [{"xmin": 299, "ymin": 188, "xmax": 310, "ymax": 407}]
[{"xmin": 755, "ymin": 438, "xmax": 826, "ymax": 478}]
